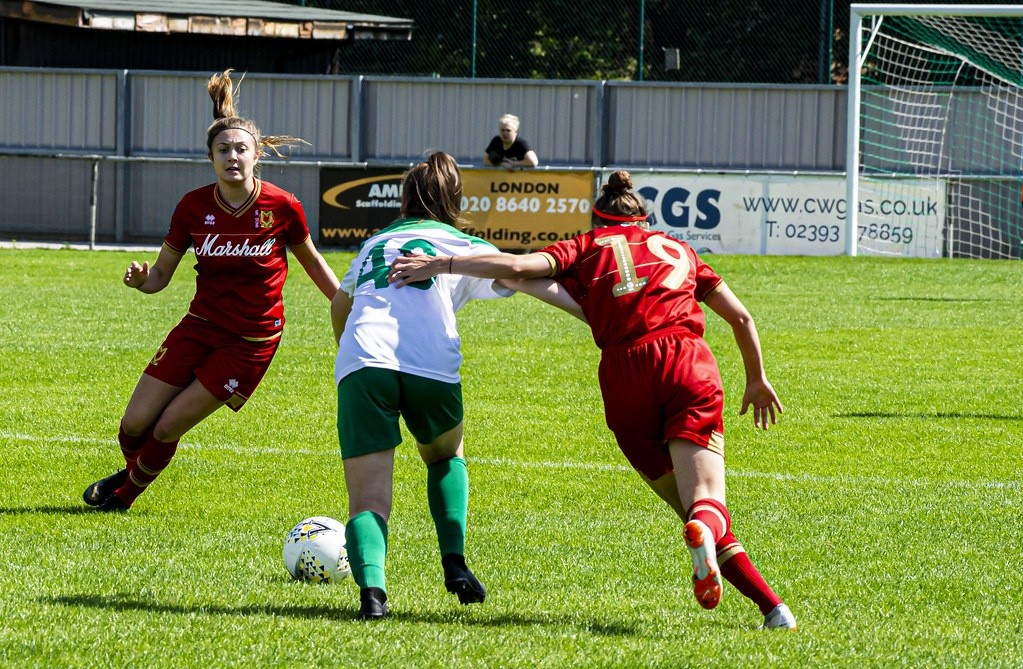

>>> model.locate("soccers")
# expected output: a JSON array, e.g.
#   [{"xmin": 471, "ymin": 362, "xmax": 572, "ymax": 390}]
[{"xmin": 282, "ymin": 517, "xmax": 352, "ymax": 584}]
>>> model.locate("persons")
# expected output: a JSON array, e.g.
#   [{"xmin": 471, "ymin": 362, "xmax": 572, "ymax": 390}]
[
  {"xmin": 388, "ymin": 170, "xmax": 797, "ymax": 631},
  {"xmin": 84, "ymin": 74, "xmax": 341, "ymax": 512},
  {"xmin": 485, "ymin": 114, "xmax": 538, "ymax": 255},
  {"xmin": 330, "ymin": 154, "xmax": 589, "ymax": 621}
]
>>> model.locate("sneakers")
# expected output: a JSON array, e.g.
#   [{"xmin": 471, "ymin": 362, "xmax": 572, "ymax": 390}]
[
  {"xmin": 358, "ymin": 588, "xmax": 388, "ymax": 619},
  {"xmin": 442, "ymin": 553, "xmax": 486, "ymax": 605}
]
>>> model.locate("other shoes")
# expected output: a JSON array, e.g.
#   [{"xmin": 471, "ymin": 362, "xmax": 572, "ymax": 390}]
[
  {"xmin": 83, "ymin": 468, "xmax": 131, "ymax": 514},
  {"xmin": 682, "ymin": 520, "xmax": 722, "ymax": 609},
  {"xmin": 757, "ymin": 603, "xmax": 797, "ymax": 632}
]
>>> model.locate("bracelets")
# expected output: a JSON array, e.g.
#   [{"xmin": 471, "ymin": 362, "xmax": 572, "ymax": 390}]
[{"xmin": 449, "ymin": 255, "xmax": 453, "ymax": 273}]
